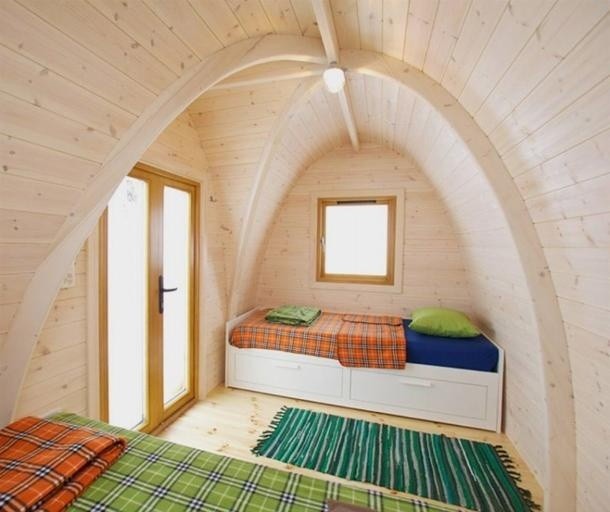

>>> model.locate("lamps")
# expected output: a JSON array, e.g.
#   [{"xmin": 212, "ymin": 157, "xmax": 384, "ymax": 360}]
[{"xmin": 322, "ymin": 61, "xmax": 349, "ymax": 94}]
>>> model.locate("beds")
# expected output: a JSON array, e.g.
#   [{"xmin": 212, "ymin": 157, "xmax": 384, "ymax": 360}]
[
  {"xmin": 223, "ymin": 304, "xmax": 506, "ymax": 435},
  {"xmin": 1, "ymin": 408, "xmax": 483, "ymax": 512}
]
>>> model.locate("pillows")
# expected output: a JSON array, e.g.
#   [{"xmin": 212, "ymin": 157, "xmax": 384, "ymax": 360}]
[{"xmin": 408, "ymin": 308, "xmax": 482, "ymax": 340}]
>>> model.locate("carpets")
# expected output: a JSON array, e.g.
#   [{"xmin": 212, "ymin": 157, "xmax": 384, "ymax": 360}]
[{"xmin": 249, "ymin": 404, "xmax": 542, "ymax": 511}]
